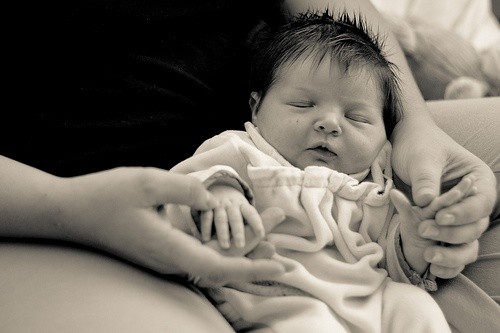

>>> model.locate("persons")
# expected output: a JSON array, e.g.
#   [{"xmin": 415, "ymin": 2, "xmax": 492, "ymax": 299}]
[
  {"xmin": 0, "ymin": 0, "xmax": 499, "ymax": 333},
  {"xmin": 159, "ymin": 3, "xmax": 478, "ymax": 333}
]
